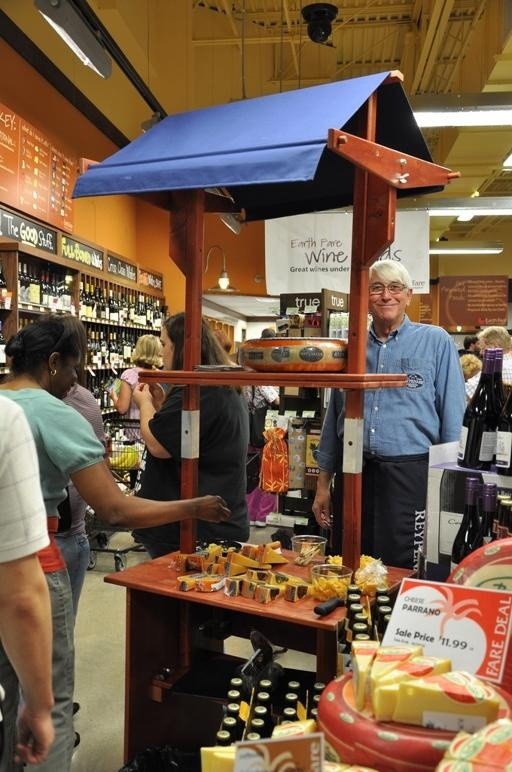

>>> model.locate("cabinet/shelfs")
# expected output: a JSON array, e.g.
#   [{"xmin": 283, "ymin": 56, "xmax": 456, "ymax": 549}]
[
  {"xmin": 0, "ymin": 239, "xmax": 167, "ymax": 423},
  {"xmin": 104, "ymin": 544, "xmax": 405, "ymax": 772}
]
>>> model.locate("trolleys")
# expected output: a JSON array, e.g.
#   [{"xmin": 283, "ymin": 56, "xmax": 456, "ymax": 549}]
[{"xmin": 83, "ymin": 406, "xmax": 148, "ymax": 571}]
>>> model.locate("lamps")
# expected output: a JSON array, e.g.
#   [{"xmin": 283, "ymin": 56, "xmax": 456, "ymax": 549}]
[
  {"xmin": 35, "ymin": 0, "xmax": 111, "ymax": 79},
  {"xmin": 203, "ymin": 245, "xmax": 240, "ymax": 294}
]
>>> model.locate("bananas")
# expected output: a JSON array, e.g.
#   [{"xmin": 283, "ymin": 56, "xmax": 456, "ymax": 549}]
[{"xmin": 108, "ymin": 446, "xmax": 139, "ymax": 468}]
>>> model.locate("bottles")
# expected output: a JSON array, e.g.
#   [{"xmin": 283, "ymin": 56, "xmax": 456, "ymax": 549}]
[
  {"xmin": 448, "ymin": 477, "xmax": 511, "ymax": 573},
  {"xmin": 84, "ymin": 324, "xmax": 160, "ymax": 366},
  {"xmin": 18, "ymin": 264, "xmax": 171, "ymax": 331},
  {"xmin": 0, "ymin": 320, "xmax": 9, "ymax": 367},
  {"xmin": 104, "ymin": 422, "xmax": 126, "ymax": 459},
  {"xmin": 0, "ymin": 266, "xmax": 9, "ymax": 308},
  {"xmin": 339, "ymin": 582, "xmax": 392, "ymax": 675},
  {"xmin": 457, "ymin": 349, "xmax": 511, "ymax": 474},
  {"xmin": 215, "ymin": 676, "xmax": 327, "ymax": 744},
  {"xmin": 88, "ymin": 372, "xmax": 116, "ymax": 409}
]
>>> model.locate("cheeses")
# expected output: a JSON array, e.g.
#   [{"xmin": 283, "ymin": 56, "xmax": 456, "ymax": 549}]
[
  {"xmin": 200, "ymin": 720, "xmax": 379, "ymax": 771},
  {"xmin": 176, "ymin": 544, "xmax": 314, "ymax": 604},
  {"xmin": 351, "ymin": 640, "xmax": 500, "ymax": 733}
]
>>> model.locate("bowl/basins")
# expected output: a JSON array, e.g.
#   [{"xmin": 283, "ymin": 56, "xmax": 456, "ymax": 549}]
[
  {"xmin": 311, "ymin": 564, "xmax": 353, "ymax": 601},
  {"xmin": 291, "ymin": 534, "xmax": 327, "ymax": 566}
]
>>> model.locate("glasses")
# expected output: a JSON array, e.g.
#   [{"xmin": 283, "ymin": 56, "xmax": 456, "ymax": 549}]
[{"xmin": 370, "ymin": 283, "xmax": 406, "ymax": 295}]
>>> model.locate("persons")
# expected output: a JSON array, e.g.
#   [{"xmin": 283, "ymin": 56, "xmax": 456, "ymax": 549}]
[
  {"xmin": 0, "ymin": 321, "xmax": 232, "ymax": 772},
  {"xmin": 0, "ymin": 396, "xmax": 56, "ymax": 772},
  {"xmin": 211, "ymin": 329, "xmax": 280, "ymax": 527},
  {"xmin": 131, "ymin": 312, "xmax": 250, "ymax": 655},
  {"xmin": 108, "ymin": 334, "xmax": 164, "ymax": 490},
  {"xmin": 36, "ymin": 314, "xmax": 106, "ymax": 750},
  {"xmin": 457, "ymin": 326, "xmax": 512, "ymax": 408},
  {"xmin": 311, "ymin": 260, "xmax": 466, "ymax": 569}
]
